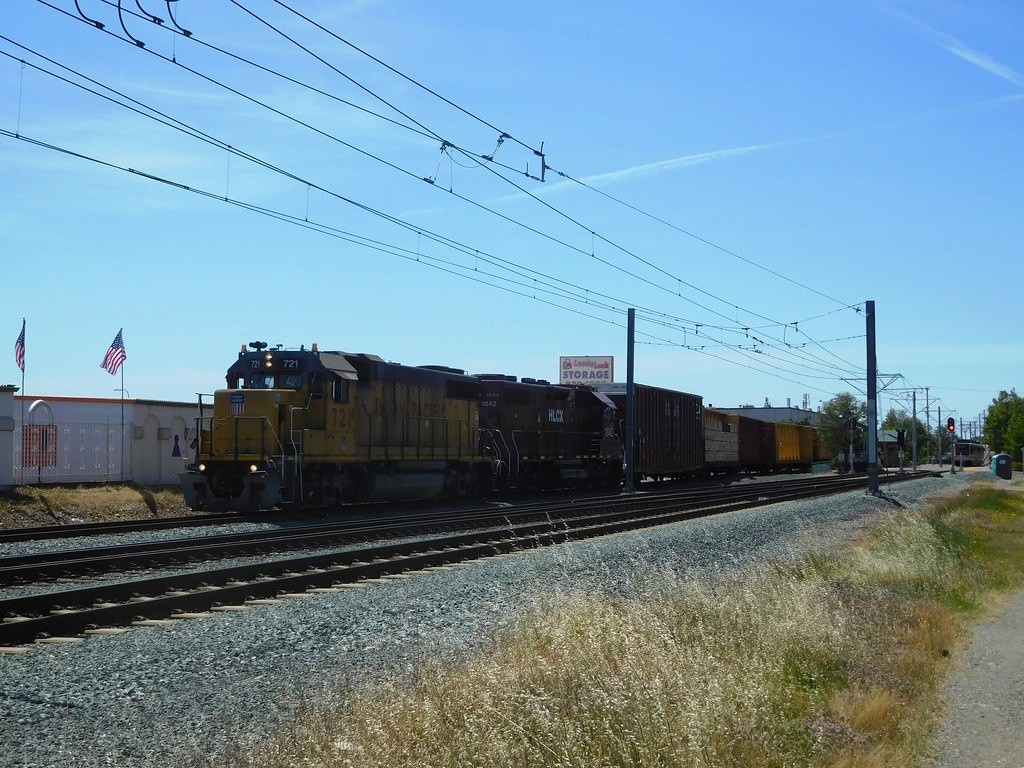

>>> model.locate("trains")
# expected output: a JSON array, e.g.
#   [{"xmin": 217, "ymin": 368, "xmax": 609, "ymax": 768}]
[
  {"xmin": 954, "ymin": 440, "xmax": 985, "ymax": 465},
  {"xmin": 177, "ymin": 340, "xmax": 839, "ymax": 519}
]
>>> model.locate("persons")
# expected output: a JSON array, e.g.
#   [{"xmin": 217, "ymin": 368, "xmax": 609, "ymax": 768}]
[{"xmin": 989, "ymin": 454, "xmax": 992, "ymax": 465}]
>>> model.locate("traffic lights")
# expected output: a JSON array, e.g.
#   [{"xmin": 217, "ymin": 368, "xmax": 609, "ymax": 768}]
[{"xmin": 948, "ymin": 418, "xmax": 954, "ymax": 431}]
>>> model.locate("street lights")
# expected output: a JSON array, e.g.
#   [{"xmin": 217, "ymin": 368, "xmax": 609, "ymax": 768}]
[{"xmin": 113, "ymin": 388, "xmax": 130, "ymax": 398}]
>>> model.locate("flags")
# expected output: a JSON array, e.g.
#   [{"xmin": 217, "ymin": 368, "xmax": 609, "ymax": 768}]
[
  {"xmin": 100, "ymin": 329, "xmax": 126, "ymax": 375},
  {"xmin": 15, "ymin": 320, "xmax": 26, "ymax": 370}
]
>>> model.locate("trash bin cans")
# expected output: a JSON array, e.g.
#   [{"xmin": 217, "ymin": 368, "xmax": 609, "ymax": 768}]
[{"xmin": 992, "ymin": 453, "xmax": 1013, "ymax": 479}]
